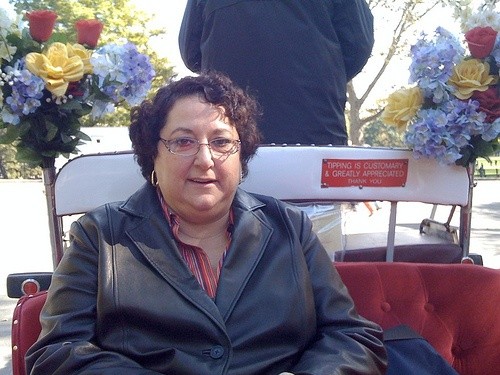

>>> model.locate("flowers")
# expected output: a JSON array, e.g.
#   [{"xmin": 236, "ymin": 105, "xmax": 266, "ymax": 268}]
[
  {"xmin": 382, "ymin": 7, "xmax": 500, "ymax": 168},
  {"xmin": 0, "ymin": 0, "xmax": 156, "ymax": 169}
]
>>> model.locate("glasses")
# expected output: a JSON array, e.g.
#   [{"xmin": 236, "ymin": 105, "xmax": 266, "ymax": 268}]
[{"xmin": 159, "ymin": 137, "xmax": 242, "ymax": 156}]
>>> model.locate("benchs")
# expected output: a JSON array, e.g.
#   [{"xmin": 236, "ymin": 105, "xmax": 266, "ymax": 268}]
[
  {"xmin": 55, "ymin": 142, "xmax": 482, "ymax": 265},
  {"xmin": 7, "ymin": 259, "xmax": 500, "ymax": 375}
]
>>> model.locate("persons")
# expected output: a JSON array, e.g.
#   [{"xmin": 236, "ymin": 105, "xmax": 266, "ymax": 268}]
[
  {"xmin": 24, "ymin": 68, "xmax": 389, "ymax": 375},
  {"xmin": 178, "ymin": 0, "xmax": 374, "ymax": 262}
]
